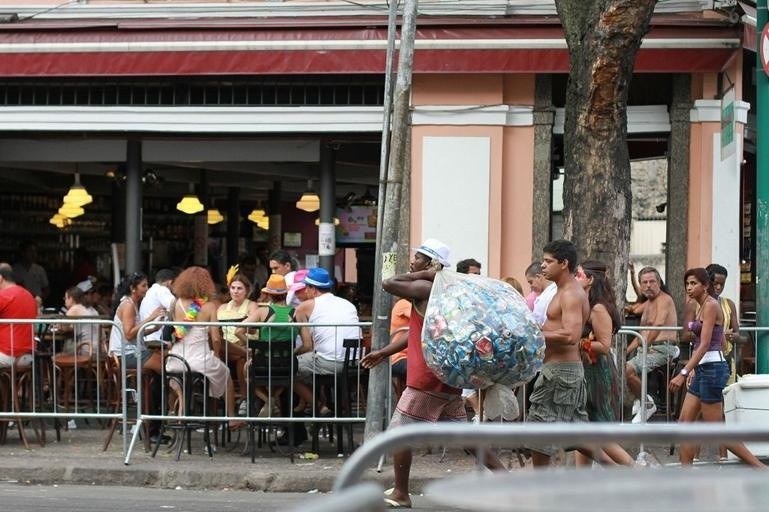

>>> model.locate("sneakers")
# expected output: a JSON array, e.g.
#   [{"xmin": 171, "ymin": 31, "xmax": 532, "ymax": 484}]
[
  {"xmin": 273, "ymin": 432, "xmax": 302, "ymax": 446},
  {"xmin": 284, "ymin": 429, "xmax": 308, "ymax": 440},
  {"xmin": 632, "ymin": 402, "xmax": 658, "ymax": 424},
  {"xmin": 166, "ymin": 392, "xmax": 348, "ymax": 431},
  {"xmin": 632, "ymin": 394, "xmax": 655, "ymax": 416}
]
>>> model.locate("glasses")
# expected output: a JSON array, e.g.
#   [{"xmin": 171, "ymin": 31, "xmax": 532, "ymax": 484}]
[{"xmin": 575, "ymin": 271, "xmax": 587, "ymax": 280}]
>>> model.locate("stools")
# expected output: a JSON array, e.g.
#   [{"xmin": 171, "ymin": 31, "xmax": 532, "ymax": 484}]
[{"xmin": 647, "ymin": 358, "xmax": 690, "ymax": 423}]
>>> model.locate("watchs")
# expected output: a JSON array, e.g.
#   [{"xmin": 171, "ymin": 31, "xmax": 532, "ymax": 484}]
[{"xmin": 680, "ymin": 367, "xmax": 689, "ymax": 377}]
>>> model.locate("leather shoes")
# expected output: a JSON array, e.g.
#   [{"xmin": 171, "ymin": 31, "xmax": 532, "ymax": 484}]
[
  {"xmin": 156, "ymin": 432, "xmax": 171, "ymax": 440},
  {"xmin": 149, "ymin": 435, "xmax": 171, "ymax": 444}
]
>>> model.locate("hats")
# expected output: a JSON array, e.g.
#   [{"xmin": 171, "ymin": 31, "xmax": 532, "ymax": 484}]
[
  {"xmin": 411, "ymin": 239, "xmax": 451, "ymax": 267},
  {"xmin": 301, "ymin": 267, "xmax": 334, "ymax": 289},
  {"xmin": 261, "ymin": 274, "xmax": 290, "ymax": 295},
  {"xmin": 292, "ymin": 270, "xmax": 308, "ymax": 292}
]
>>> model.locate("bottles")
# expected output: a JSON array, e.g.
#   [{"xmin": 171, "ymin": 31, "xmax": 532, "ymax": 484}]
[{"xmin": 0, "ymin": 193, "xmax": 195, "ymax": 272}]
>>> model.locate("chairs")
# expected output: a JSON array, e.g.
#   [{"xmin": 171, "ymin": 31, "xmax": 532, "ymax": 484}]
[
  {"xmin": 51, "ymin": 341, "xmax": 103, "ymax": 430},
  {"xmin": 1, "ymin": 350, "xmax": 42, "ymax": 450},
  {"xmin": 102, "ymin": 340, "xmax": 142, "ymax": 439},
  {"xmin": 23, "ymin": 318, "xmax": 62, "ymax": 426},
  {"xmin": 246, "ymin": 338, "xmax": 299, "ymax": 463},
  {"xmin": 103, "ymin": 350, "xmax": 154, "ymax": 452},
  {"xmin": 151, "ymin": 354, "xmax": 214, "ymax": 457},
  {"xmin": 311, "ymin": 338, "xmax": 370, "ymax": 456}
]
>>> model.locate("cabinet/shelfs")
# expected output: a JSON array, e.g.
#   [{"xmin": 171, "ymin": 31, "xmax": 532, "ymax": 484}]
[
  {"xmin": 0, "ymin": 193, "xmax": 113, "ymax": 281},
  {"xmin": 143, "ymin": 194, "xmax": 270, "ymax": 237}
]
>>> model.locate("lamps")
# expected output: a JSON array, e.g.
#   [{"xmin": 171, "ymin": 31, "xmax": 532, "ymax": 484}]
[
  {"xmin": 57, "ymin": 204, "xmax": 85, "ymax": 218},
  {"xmin": 176, "ymin": 182, "xmax": 205, "ymax": 215},
  {"xmin": 207, "ymin": 195, "xmax": 224, "ymax": 225},
  {"xmin": 248, "ymin": 200, "xmax": 265, "ymax": 222},
  {"xmin": 257, "ymin": 216, "xmax": 269, "ymax": 231},
  {"xmin": 315, "ymin": 217, "xmax": 340, "ymax": 226},
  {"xmin": 63, "ymin": 162, "xmax": 93, "ymax": 205},
  {"xmin": 48, "ymin": 213, "xmax": 72, "ymax": 229},
  {"xmin": 295, "ymin": 179, "xmax": 320, "ymax": 212}
]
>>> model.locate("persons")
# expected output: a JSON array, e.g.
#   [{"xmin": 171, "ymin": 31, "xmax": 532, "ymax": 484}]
[
  {"xmin": 1, "ymin": 241, "xmax": 679, "ymax": 446},
  {"xmin": 670, "ymin": 269, "xmax": 768, "ymax": 470},
  {"xmin": 683, "ymin": 263, "xmax": 740, "ymax": 461},
  {"xmin": 292, "ymin": 268, "xmax": 358, "ymax": 417},
  {"xmin": 571, "ymin": 260, "xmax": 644, "ymax": 471},
  {"xmin": 357, "ymin": 239, "xmax": 511, "ymax": 509},
  {"xmin": 526, "ymin": 240, "xmax": 619, "ymax": 473}
]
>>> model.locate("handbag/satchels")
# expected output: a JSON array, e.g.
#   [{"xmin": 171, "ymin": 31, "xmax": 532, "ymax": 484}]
[{"xmin": 160, "ymin": 325, "xmax": 175, "ymax": 341}]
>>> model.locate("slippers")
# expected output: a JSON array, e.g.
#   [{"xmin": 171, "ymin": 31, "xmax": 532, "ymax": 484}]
[{"xmin": 383, "ymin": 497, "xmax": 412, "ymax": 511}]
